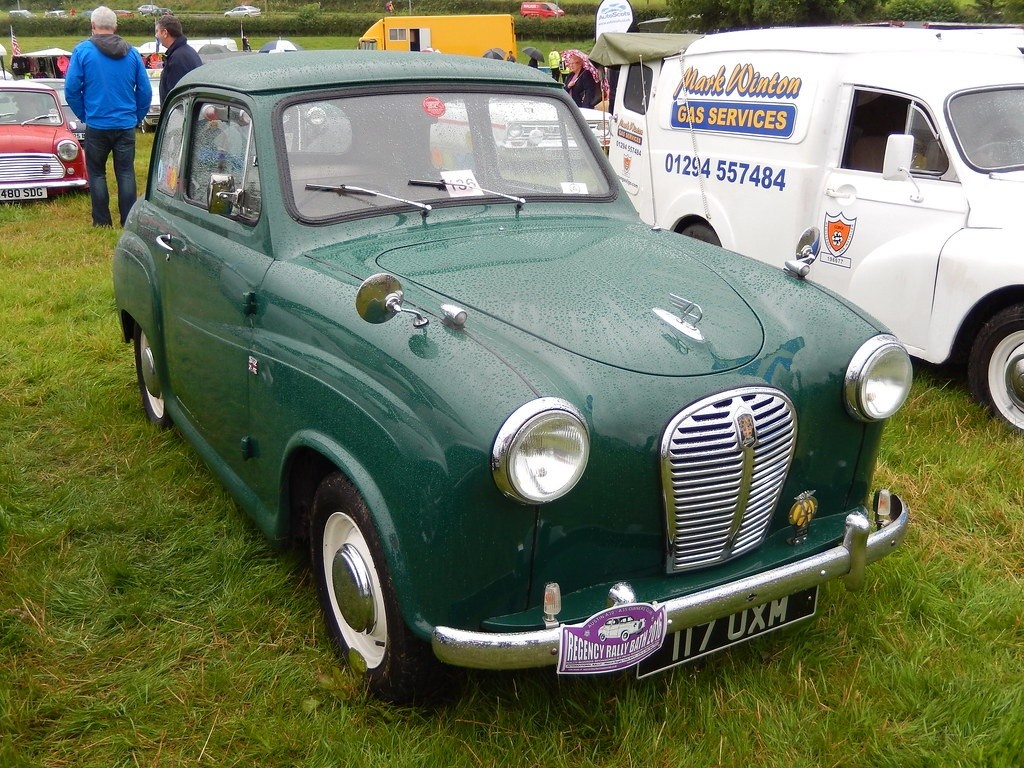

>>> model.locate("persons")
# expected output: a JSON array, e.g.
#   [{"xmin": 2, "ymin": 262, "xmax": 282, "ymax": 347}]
[
  {"xmin": 529, "ymin": 57, "xmax": 538, "ymax": 68},
  {"xmin": 561, "ymin": 54, "xmax": 570, "ymax": 83},
  {"xmin": 507, "ymin": 51, "xmax": 515, "ymax": 62},
  {"xmin": 66, "ymin": 6, "xmax": 152, "ymax": 228},
  {"xmin": 242, "ymin": 33, "xmax": 250, "ymax": 50},
  {"xmin": 39, "ymin": 68, "xmax": 48, "ymax": 78},
  {"xmin": 564, "ymin": 55, "xmax": 602, "ymax": 109},
  {"xmin": 156, "ymin": 14, "xmax": 204, "ymax": 112},
  {"xmin": 549, "ymin": 47, "xmax": 560, "ymax": 81}
]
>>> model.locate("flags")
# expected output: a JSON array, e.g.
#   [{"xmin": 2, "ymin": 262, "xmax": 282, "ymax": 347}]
[
  {"xmin": 155, "ymin": 22, "xmax": 160, "ymax": 52},
  {"xmin": 11, "ymin": 31, "xmax": 20, "ymax": 55}
]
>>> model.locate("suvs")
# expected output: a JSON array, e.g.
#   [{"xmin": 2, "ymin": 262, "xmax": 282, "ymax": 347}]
[{"xmin": 137, "ymin": 4, "xmax": 170, "ymax": 18}]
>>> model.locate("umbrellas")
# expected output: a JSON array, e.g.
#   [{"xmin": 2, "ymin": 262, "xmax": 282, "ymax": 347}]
[
  {"xmin": 258, "ymin": 39, "xmax": 302, "ymax": 52},
  {"xmin": 559, "ymin": 50, "xmax": 610, "ymax": 101},
  {"xmin": 421, "ymin": 47, "xmax": 439, "ymax": 52},
  {"xmin": 483, "ymin": 48, "xmax": 505, "ymax": 60},
  {"xmin": 522, "ymin": 47, "xmax": 545, "ymax": 63}
]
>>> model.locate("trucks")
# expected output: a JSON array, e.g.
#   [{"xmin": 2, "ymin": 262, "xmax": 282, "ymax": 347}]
[{"xmin": 357, "ymin": 13, "xmax": 518, "ymax": 63}]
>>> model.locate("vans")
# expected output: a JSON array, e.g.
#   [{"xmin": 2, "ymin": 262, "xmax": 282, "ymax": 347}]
[
  {"xmin": 520, "ymin": 2, "xmax": 565, "ymax": 19},
  {"xmin": 606, "ymin": 20, "xmax": 1024, "ymax": 437},
  {"xmin": 8, "ymin": 10, "xmax": 33, "ymax": 20}
]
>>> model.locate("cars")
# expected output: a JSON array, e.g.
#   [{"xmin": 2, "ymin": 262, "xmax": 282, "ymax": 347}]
[
  {"xmin": 114, "ymin": 49, "xmax": 916, "ymax": 714},
  {"xmin": 224, "ymin": 5, "xmax": 262, "ymax": 19},
  {"xmin": 45, "ymin": 10, "xmax": 67, "ymax": 18},
  {"xmin": 14, "ymin": 79, "xmax": 86, "ymax": 148},
  {"xmin": 0, "ymin": 79, "xmax": 91, "ymax": 202},
  {"xmin": 114, "ymin": 9, "xmax": 134, "ymax": 18},
  {"xmin": 137, "ymin": 77, "xmax": 162, "ymax": 134},
  {"xmin": 429, "ymin": 96, "xmax": 614, "ymax": 170}
]
